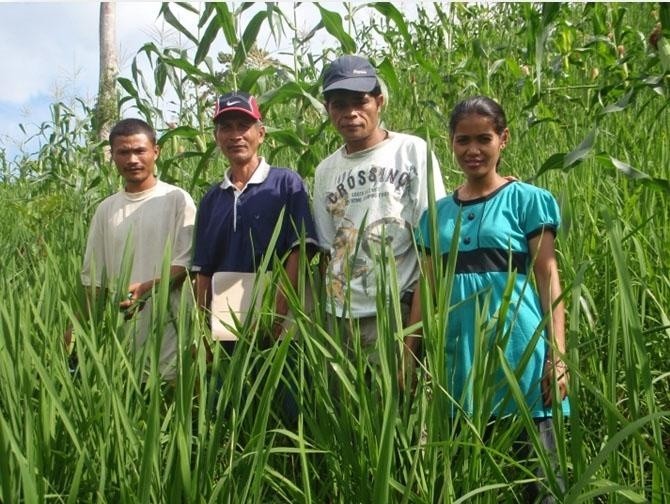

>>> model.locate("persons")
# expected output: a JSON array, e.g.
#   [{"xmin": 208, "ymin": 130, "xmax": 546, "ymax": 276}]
[
  {"xmin": 397, "ymin": 95, "xmax": 569, "ymax": 504},
  {"xmin": 65, "ymin": 117, "xmax": 197, "ymax": 407},
  {"xmin": 313, "ymin": 54, "xmax": 447, "ymax": 405},
  {"xmin": 192, "ymin": 90, "xmax": 318, "ymax": 449}
]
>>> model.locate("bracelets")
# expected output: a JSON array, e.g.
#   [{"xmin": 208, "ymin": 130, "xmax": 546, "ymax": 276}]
[{"xmin": 546, "ymin": 359, "xmax": 567, "ymax": 368}]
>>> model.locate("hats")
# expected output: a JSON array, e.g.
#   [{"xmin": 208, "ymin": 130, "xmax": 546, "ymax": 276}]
[
  {"xmin": 213, "ymin": 92, "xmax": 261, "ymax": 121},
  {"xmin": 321, "ymin": 55, "xmax": 380, "ymax": 94}
]
